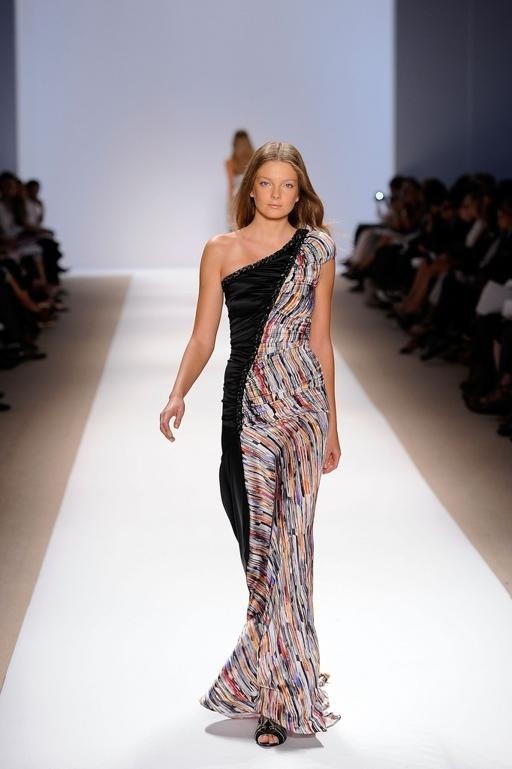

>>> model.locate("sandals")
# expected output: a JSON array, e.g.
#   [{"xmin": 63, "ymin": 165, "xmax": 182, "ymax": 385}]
[{"xmin": 255, "ymin": 716, "xmax": 287, "ymax": 748}]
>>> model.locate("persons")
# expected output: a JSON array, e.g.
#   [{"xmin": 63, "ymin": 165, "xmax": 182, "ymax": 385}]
[
  {"xmin": 341, "ymin": 170, "xmax": 511, "ymax": 441},
  {"xmin": 0, "ymin": 173, "xmax": 71, "ymax": 411},
  {"xmin": 160, "ymin": 142, "xmax": 341, "ymax": 748},
  {"xmin": 226, "ymin": 132, "xmax": 257, "ymax": 231}
]
